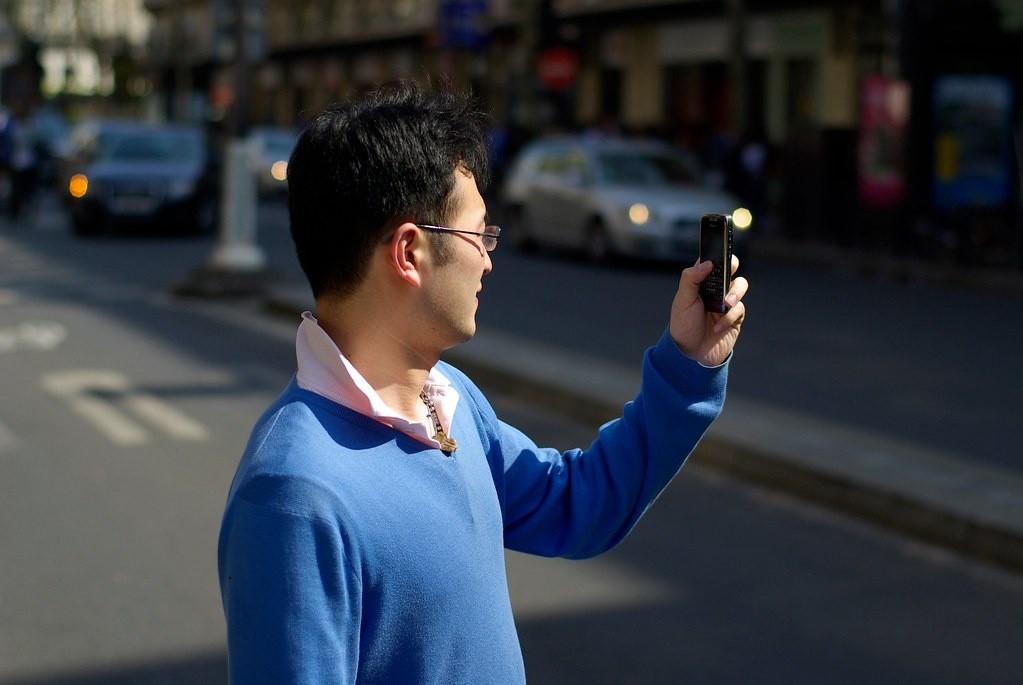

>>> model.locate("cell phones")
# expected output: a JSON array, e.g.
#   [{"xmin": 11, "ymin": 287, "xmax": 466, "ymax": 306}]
[{"xmin": 700, "ymin": 213, "xmax": 734, "ymax": 313}]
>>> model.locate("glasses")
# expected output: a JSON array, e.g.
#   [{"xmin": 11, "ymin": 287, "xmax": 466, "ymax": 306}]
[{"xmin": 384, "ymin": 225, "xmax": 504, "ymax": 252}]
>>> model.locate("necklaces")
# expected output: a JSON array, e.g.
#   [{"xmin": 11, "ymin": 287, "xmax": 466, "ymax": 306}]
[{"xmin": 419, "ymin": 391, "xmax": 458, "ymax": 453}]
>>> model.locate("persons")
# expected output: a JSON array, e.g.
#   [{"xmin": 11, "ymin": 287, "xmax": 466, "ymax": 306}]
[
  {"xmin": 0, "ymin": 92, "xmax": 55, "ymax": 217},
  {"xmin": 217, "ymin": 67, "xmax": 748, "ymax": 685}
]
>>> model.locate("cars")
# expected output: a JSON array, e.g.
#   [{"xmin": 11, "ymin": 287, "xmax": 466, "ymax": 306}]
[
  {"xmin": 242, "ymin": 126, "xmax": 299, "ymax": 206},
  {"xmin": 52, "ymin": 116, "xmax": 216, "ymax": 237},
  {"xmin": 498, "ymin": 131, "xmax": 758, "ymax": 271}
]
